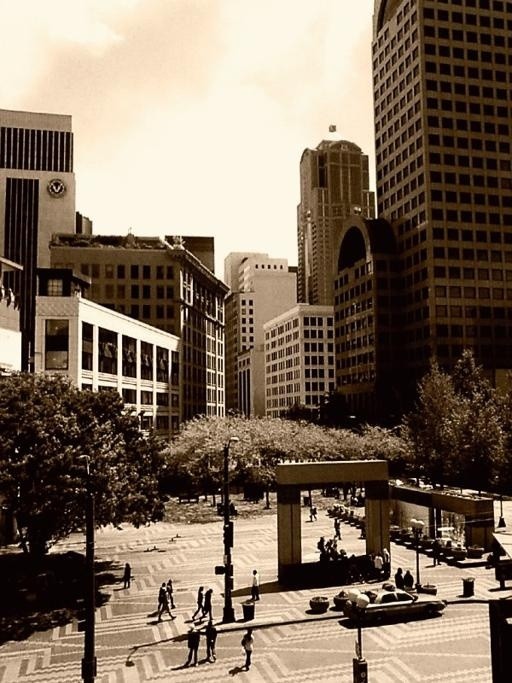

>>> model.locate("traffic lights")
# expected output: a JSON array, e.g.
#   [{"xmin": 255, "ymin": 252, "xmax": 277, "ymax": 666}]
[{"xmin": 223, "ymin": 522, "xmax": 233, "ymax": 547}]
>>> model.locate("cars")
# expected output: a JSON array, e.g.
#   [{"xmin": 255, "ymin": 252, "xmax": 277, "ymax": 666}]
[{"xmin": 343, "ymin": 583, "xmax": 446, "ymax": 622}]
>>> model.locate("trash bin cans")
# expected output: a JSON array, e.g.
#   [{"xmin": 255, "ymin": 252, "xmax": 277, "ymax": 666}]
[
  {"xmin": 304, "ymin": 497, "xmax": 309, "ymax": 506},
  {"xmin": 243, "ymin": 602, "xmax": 254, "ymax": 620},
  {"xmin": 462, "ymin": 577, "xmax": 474, "ymax": 596}
]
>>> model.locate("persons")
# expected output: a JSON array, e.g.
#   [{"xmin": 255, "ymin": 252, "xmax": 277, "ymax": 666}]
[
  {"xmin": 157, "ymin": 585, "xmax": 176, "ymax": 621},
  {"xmin": 394, "ymin": 567, "xmax": 404, "ymax": 590},
  {"xmin": 432, "ymin": 539, "xmax": 441, "ymax": 567},
  {"xmin": 309, "ymin": 505, "xmax": 317, "ymax": 521},
  {"xmin": 123, "ymin": 562, "xmax": 131, "ymax": 588},
  {"xmin": 241, "ymin": 628, "xmax": 254, "ymax": 671},
  {"xmin": 205, "ymin": 621, "xmax": 218, "ymax": 662},
  {"xmin": 198, "ymin": 588, "xmax": 215, "ymax": 623},
  {"xmin": 166, "ymin": 579, "xmax": 177, "ymax": 609},
  {"xmin": 333, "ymin": 518, "xmax": 341, "ymax": 540},
  {"xmin": 404, "ymin": 570, "xmax": 413, "ymax": 589},
  {"xmin": 250, "ymin": 569, "xmax": 260, "ymax": 601},
  {"xmin": 191, "ymin": 586, "xmax": 208, "ymax": 620},
  {"xmin": 378, "ymin": 548, "xmax": 390, "ymax": 571},
  {"xmin": 374, "ymin": 550, "xmax": 388, "ymax": 581},
  {"xmin": 157, "ymin": 581, "xmax": 169, "ymax": 614},
  {"xmin": 182, "ymin": 623, "xmax": 200, "ymax": 667}
]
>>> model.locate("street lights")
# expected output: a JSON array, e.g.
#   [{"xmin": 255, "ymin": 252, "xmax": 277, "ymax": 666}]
[
  {"xmin": 223, "ymin": 437, "xmax": 239, "ymax": 622},
  {"xmin": 75, "ymin": 455, "xmax": 97, "ymax": 683},
  {"xmin": 410, "ymin": 518, "xmax": 425, "ymax": 593},
  {"xmin": 348, "ymin": 588, "xmax": 370, "ymax": 683}
]
